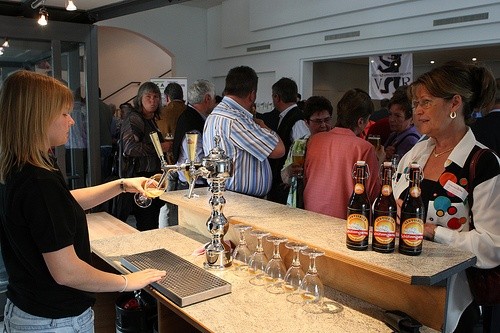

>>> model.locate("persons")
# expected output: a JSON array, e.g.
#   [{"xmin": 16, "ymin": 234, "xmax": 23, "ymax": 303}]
[
  {"xmin": 203, "ymin": 66, "xmax": 285, "ymax": 198},
  {"xmin": 0, "ymin": 72, "xmax": 167, "ymax": 333},
  {"xmin": 371, "ymin": 61, "xmax": 500, "ymax": 333},
  {"xmin": 254, "ymin": 77, "xmax": 332, "ymax": 207},
  {"xmin": 60, "ymin": 79, "xmax": 215, "ymax": 231},
  {"xmin": 301, "ymin": 88, "xmax": 385, "ymax": 220}
]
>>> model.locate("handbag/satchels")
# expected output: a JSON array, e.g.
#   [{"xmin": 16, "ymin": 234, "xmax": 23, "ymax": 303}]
[{"xmin": 118, "ymin": 121, "xmax": 161, "ymax": 178}]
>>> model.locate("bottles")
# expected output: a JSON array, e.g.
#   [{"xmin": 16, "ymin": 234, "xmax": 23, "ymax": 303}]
[
  {"xmin": 370, "ymin": 162, "xmax": 397, "ymax": 254},
  {"xmin": 346, "ymin": 160, "xmax": 370, "ymax": 251},
  {"xmin": 397, "ymin": 163, "xmax": 425, "ymax": 256}
]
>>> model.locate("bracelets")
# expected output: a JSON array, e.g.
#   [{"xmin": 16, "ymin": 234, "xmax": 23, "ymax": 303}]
[
  {"xmin": 121, "ymin": 179, "xmax": 123, "ymax": 190},
  {"xmin": 119, "ymin": 275, "xmax": 127, "ymax": 293}
]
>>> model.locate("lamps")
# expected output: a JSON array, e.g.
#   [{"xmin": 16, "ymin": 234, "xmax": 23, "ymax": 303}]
[
  {"xmin": 66, "ymin": 0, "xmax": 77, "ymax": 11},
  {"xmin": 37, "ymin": 0, "xmax": 48, "ymax": 26}
]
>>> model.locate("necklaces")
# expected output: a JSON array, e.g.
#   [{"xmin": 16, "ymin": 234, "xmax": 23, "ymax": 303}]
[{"xmin": 433, "ymin": 147, "xmax": 453, "ymax": 157}]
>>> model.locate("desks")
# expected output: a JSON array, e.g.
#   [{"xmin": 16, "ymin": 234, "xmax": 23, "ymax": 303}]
[{"xmin": 86, "ymin": 211, "xmax": 140, "ymax": 241}]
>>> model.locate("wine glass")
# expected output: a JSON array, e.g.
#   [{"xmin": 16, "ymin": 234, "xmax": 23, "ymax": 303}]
[
  {"xmin": 281, "ymin": 242, "xmax": 308, "ymax": 303},
  {"xmin": 296, "ymin": 247, "xmax": 324, "ymax": 307},
  {"xmin": 134, "ymin": 173, "xmax": 168, "ymax": 208},
  {"xmin": 246, "ymin": 228, "xmax": 270, "ymax": 286},
  {"xmin": 261, "ymin": 236, "xmax": 289, "ymax": 294},
  {"xmin": 230, "ymin": 224, "xmax": 252, "ymax": 276}
]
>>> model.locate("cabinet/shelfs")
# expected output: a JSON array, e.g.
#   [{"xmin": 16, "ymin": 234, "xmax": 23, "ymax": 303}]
[{"xmin": 87, "ymin": 186, "xmax": 476, "ymax": 333}]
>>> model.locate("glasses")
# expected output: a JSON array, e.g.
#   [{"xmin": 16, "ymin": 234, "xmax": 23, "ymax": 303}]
[
  {"xmin": 408, "ymin": 96, "xmax": 436, "ymax": 109},
  {"xmin": 386, "ymin": 110, "xmax": 405, "ymax": 119},
  {"xmin": 306, "ymin": 116, "xmax": 331, "ymax": 125}
]
